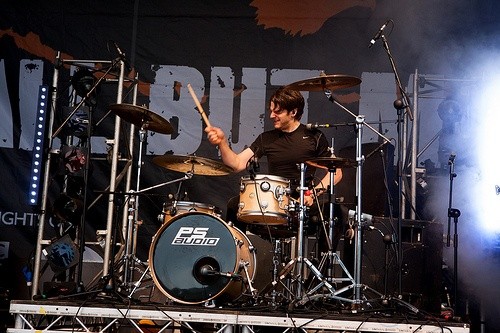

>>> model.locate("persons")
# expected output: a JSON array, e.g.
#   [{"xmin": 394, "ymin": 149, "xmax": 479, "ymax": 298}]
[{"xmin": 205, "ymin": 85, "xmax": 348, "ymax": 301}]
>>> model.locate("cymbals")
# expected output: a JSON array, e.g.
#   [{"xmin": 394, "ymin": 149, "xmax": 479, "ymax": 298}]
[
  {"xmin": 151, "ymin": 154, "xmax": 234, "ymax": 176},
  {"xmin": 285, "ymin": 74, "xmax": 362, "ymax": 92},
  {"xmin": 108, "ymin": 103, "xmax": 175, "ymax": 135},
  {"xmin": 305, "ymin": 157, "xmax": 359, "ymax": 170}
]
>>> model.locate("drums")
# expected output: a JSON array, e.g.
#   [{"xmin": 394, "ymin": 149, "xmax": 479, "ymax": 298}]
[
  {"xmin": 159, "ymin": 200, "xmax": 215, "ymax": 224},
  {"xmin": 146, "ymin": 209, "xmax": 257, "ymax": 307},
  {"xmin": 238, "ymin": 174, "xmax": 291, "ymax": 226}
]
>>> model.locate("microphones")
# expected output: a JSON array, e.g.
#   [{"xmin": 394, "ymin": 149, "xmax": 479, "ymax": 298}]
[
  {"xmin": 448, "ymin": 153, "xmax": 456, "ymax": 165},
  {"xmin": 368, "ymin": 20, "xmax": 389, "ymax": 48},
  {"xmin": 193, "ymin": 256, "xmax": 221, "ymax": 285},
  {"xmin": 306, "ymin": 123, "xmax": 333, "ymax": 129}
]
